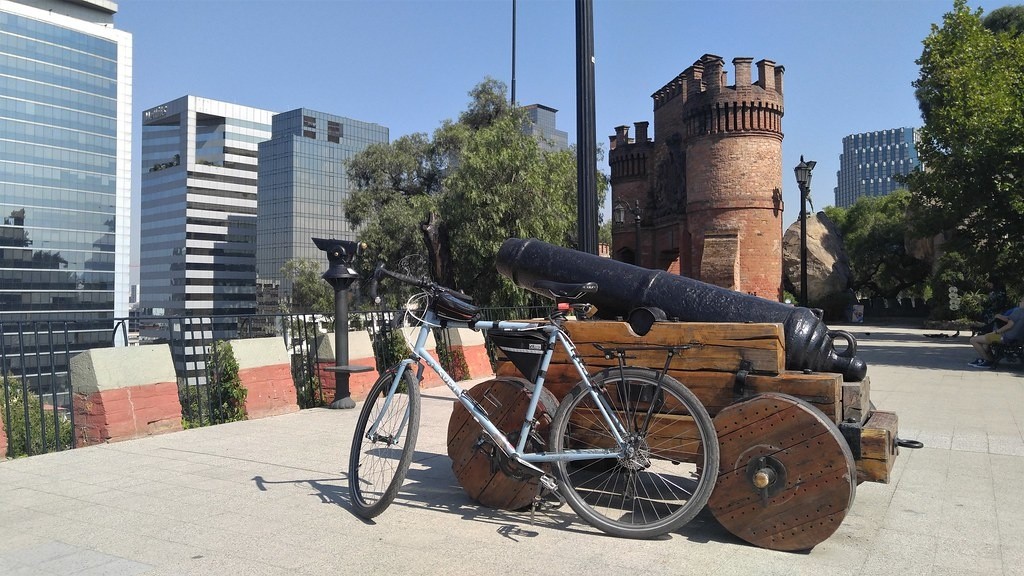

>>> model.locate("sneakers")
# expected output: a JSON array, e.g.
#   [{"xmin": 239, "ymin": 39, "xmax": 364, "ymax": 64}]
[
  {"xmin": 972, "ymin": 360, "xmax": 993, "ymax": 368},
  {"xmin": 967, "ymin": 357, "xmax": 984, "ymax": 366}
]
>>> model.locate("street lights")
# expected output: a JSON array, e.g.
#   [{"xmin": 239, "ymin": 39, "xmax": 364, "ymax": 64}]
[{"xmin": 793, "ymin": 154, "xmax": 809, "ymax": 306}]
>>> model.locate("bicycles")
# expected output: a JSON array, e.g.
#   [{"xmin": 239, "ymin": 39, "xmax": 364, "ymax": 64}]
[{"xmin": 349, "ymin": 241, "xmax": 719, "ymax": 539}]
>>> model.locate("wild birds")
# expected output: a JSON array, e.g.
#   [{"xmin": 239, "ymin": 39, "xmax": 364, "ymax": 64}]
[
  {"xmin": 865, "ymin": 332, "xmax": 871, "ymax": 335},
  {"xmin": 923, "ymin": 329, "xmax": 960, "ymax": 338}
]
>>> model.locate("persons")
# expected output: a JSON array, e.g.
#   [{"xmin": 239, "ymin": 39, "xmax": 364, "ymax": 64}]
[{"xmin": 967, "ymin": 293, "xmax": 1023, "ymax": 369}]
